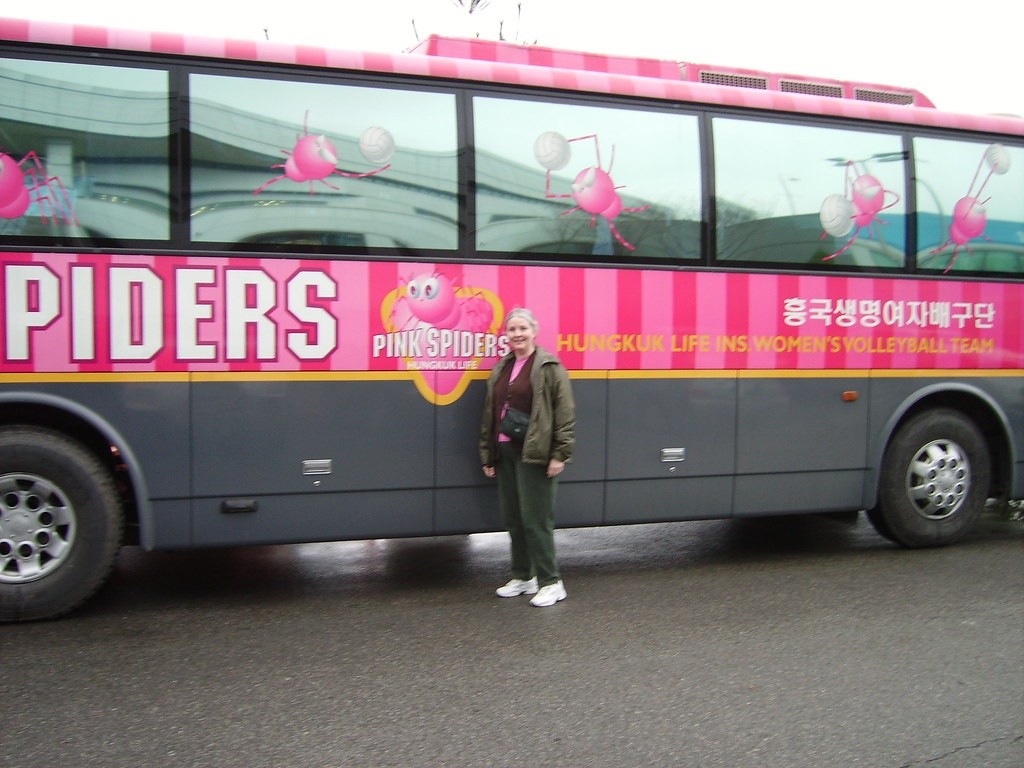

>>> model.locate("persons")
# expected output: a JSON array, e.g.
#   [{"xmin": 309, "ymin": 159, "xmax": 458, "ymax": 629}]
[{"xmin": 480, "ymin": 307, "xmax": 576, "ymax": 606}]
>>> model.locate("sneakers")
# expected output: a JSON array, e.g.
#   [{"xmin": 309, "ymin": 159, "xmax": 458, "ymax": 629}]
[
  {"xmin": 496, "ymin": 576, "xmax": 539, "ymax": 598},
  {"xmin": 529, "ymin": 577, "xmax": 567, "ymax": 606}
]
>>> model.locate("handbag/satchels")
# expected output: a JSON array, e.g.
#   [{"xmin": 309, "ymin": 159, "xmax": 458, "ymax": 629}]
[{"xmin": 499, "ymin": 407, "xmax": 530, "ymax": 443}]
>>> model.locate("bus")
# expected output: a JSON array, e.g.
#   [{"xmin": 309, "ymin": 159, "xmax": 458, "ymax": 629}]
[{"xmin": 0, "ymin": 16, "xmax": 1024, "ymax": 628}]
